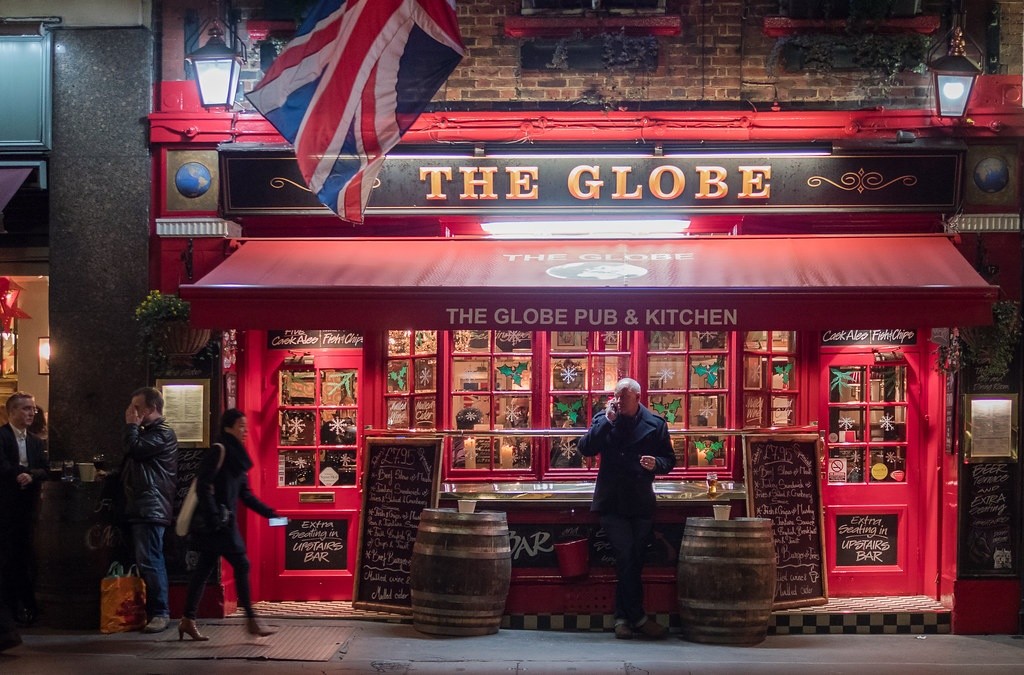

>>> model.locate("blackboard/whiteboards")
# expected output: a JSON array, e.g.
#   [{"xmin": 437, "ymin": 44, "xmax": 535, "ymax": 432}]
[
  {"xmin": 352, "ymin": 436, "xmax": 443, "ymax": 611},
  {"xmin": 162, "ymin": 448, "xmax": 218, "ymax": 585},
  {"xmin": 286, "ymin": 519, "xmax": 346, "ymax": 569},
  {"xmin": 742, "ymin": 434, "xmax": 829, "ymax": 610},
  {"xmin": 836, "ymin": 514, "xmax": 897, "ymax": 565},
  {"xmin": 960, "ymin": 463, "xmax": 1024, "ymax": 577}
]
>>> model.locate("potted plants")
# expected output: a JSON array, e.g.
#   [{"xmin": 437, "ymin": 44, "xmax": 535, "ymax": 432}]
[
  {"xmin": 935, "ymin": 300, "xmax": 1024, "ymax": 380},
  {"xmin": 132, "ymin": 288, "xmax": 210, "ymax": 368}
]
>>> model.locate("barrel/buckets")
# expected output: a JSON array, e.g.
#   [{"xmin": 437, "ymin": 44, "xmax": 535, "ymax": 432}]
[
  {"xmin": 552, "ymin": 537, "xmax": 589, "ymax": 577},
  {"xmin": 409, "ymin": 508, "xmax": 511, "ymax": 636},
  {"xmin": 677, "ymin": 515, "xmax": 777, "ymax": 647},
  {"xmin": 23, "ymin": 479, "xmax": 127, "ymax": 630}
]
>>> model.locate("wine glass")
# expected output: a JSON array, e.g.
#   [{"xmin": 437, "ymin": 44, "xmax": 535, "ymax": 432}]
[{"xmin": 93, "ymin": 452, "xmax": 105, "ymax": 480}]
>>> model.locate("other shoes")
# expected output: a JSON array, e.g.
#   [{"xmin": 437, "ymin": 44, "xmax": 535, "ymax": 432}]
[
  {"xmin": 0, "ymin": 623, "xmax": 22, "ymax": 651},
  {"xmin": 13, "ymin": 607, "xmax": 35, "ymax": 623},
  {"xmin": 615, "ymin": 624, "xmax": 633, "ymax": 638},
  {"xmin": 145, "ymin": 615, "xmax": 170, "ymax": 632},
  {"xmin": 636, "ymin": 619, "xmax": 669, "ymax": 636}
]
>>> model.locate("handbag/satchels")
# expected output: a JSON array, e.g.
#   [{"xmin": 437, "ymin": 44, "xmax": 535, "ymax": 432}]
[
  {"xmin": 176, "ymin": 443, "xmax": 225, "ymax": 536},
  {"xmin": 99, "ymin": 561, "xmax": 147, "ymax": 633}
]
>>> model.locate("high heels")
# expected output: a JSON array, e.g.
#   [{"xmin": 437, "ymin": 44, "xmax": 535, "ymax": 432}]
[
  {"xmin": 178, "ymin": 617, "xmax": 211, "ymax": 641},
  {"xmin": 245, "ymin": 617, "xmax": 278, "ymax": 638}
]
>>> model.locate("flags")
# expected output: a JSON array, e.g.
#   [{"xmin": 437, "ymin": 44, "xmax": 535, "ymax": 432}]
[{"xmin": 242, "ymin": 0, "xmax": 467, "ymax": 226}]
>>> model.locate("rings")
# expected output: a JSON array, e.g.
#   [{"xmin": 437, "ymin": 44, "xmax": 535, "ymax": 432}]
[
  {"xmin": 645, "ymin": 462, "xmax": 648, "ymax": 465},
  {"xmin": 646, "ymin": 466, "xmax": 649, "ymax": 469}
]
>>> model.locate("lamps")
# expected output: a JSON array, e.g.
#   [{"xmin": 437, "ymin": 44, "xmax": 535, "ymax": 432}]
[
  {"xmin": 183, "ymin": 0, "xmax": 247, "ymax": 114},
  {"xmin": 922, "ymin": 0, "xmax": 987, "ymax": 126},
  {"xmin": 847, "ymin": 371, "xmax": 862, "ymax": 400}
]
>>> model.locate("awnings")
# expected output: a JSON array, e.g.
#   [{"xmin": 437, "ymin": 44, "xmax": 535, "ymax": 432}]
[
  {"xmin": 0, "ymin": 168, "xmax": 33, "ymax": 213},
  {"xmin": 178, "ymin": 233, "xmax": 1000, "ymax": 332}
]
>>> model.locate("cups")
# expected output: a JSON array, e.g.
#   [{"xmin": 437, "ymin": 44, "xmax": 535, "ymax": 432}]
[
  {"xmin": 63, "ymin": 458, "xmax": 74, "ymax": 482},
  {"xmin": 78, "ymin": 462, "xmax": 95, "ymax": 482},
  {"xmin": 713, "ymin": 505, "xmax": 731, "ymax": 520},
  {"xmin": 458, "ymin": 500, "xmax": 477, "ymax": 513}
]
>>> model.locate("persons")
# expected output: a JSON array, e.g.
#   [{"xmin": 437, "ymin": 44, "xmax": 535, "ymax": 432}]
[
  {"xmin": 690, "ymin": 415, "xmax": 714, "ymax": 442},
  {"xmin": 94, "ymin": 386, "xmax": 178, "ymax": 633},
  {"xmin": 452, "ymin": 395, "xmax": 587, "ymax": 469},
  {"xmin": 178, "ymin": 408, "xmax": 291, "ymax": 641},
  {"xmin": 0, "ymin": 392, "xmax": 52, "ymax": 652},
  {"xmin": 576, "ymin": 378, "xmax": 677, "ymax": 640}
]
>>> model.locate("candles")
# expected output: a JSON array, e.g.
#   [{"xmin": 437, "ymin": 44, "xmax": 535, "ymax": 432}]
[
  {"xmin": 697, "ymin": 448, "xmax": 711, "ymax": 468},
  {"xmin": 464, "ymin": 438, "xmax": 475, "ymax": 469},
  {"xmin": 501, "ymin": 444, "xmax": 513, "ymax": 470}
]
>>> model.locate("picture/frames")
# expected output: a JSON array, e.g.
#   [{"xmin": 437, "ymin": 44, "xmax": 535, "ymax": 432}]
[
  {"xmin": 651, "ymin": 376, "xmax": 663, "ymax": 407},
  {"xmin": 704, "ymin": 378, "xmax": 718, "ymax": 400},
  {"xmin": 662, "ymin": 331, "xmax": 680, "ymax": 349},
  {"xmin": 38, "ymin": 336, "xmax": 50, "ymax": 375},
  {"xmin": 557, "ymin": 331, "xmax": 618, "ymax": 346},
  {"xmin": 155, "ymin": 378, "xmax": 210, "ymax": 449}
]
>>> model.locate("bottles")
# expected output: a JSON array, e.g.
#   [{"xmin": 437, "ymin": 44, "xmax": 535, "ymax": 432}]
[{"xmin": 706, "ymin": 473, "xmax": 718, "ymax": 500}]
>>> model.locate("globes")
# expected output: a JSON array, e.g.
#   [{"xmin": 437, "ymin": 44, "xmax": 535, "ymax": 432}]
[
  {"xmin": 176, "ymin": 163, "xmax": 211, "ymax": 198},
  {"xmin": 974, "ymin": 158, "xmax": 1008, "ymax": 192}
]
